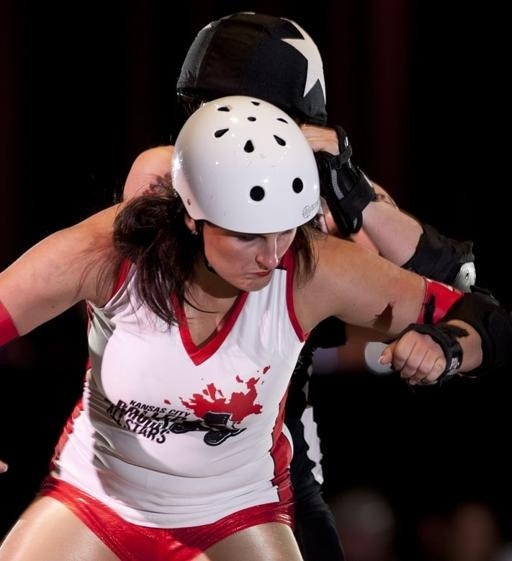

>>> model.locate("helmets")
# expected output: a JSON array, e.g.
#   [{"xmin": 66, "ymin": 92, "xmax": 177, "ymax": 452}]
[
  {"xmin": 170, "ymin": 95, "xmax": 321, "ymax": 235},
  {"xmin": 174, "ymin": 11, "xmax": 329, "ymax": 127}
]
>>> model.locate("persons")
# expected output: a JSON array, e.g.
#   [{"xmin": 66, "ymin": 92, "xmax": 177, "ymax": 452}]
[
  {"xmin": 2, "ymin": 94, "xmax": 512, "ymax": 557},
  {"xmin": 302, "ymin": 315, "xmax": 506, "ymax": 561},
  {"xmin": 116, "ymin": 11, "xmax": 493, "ymax": 557}
]
